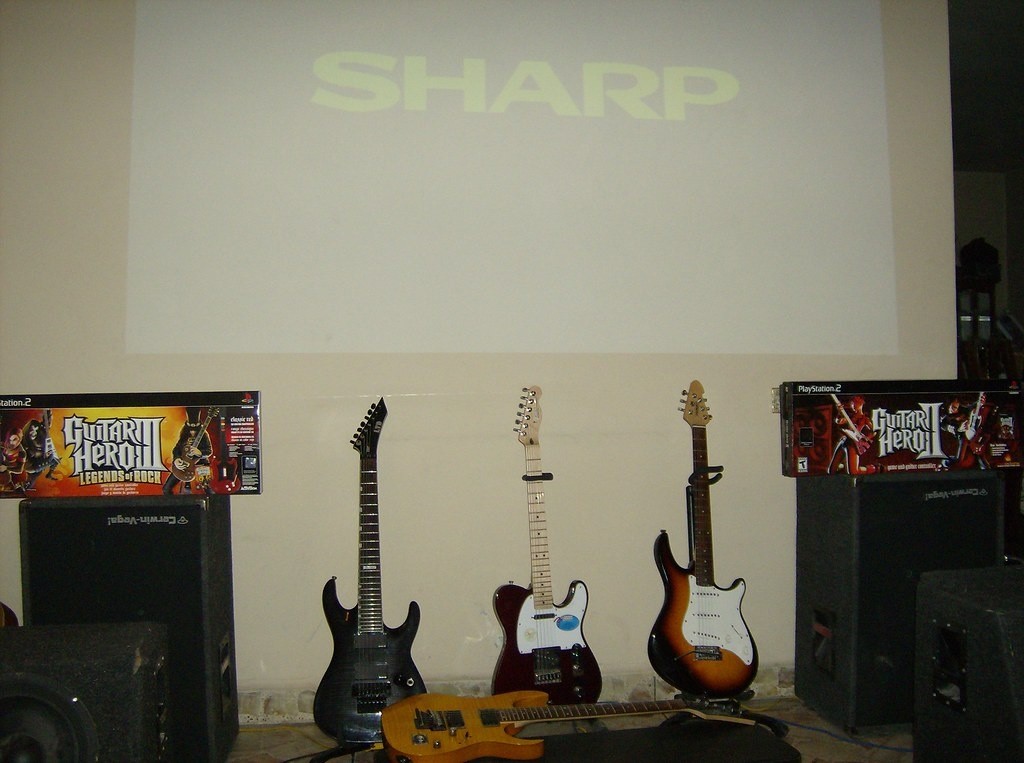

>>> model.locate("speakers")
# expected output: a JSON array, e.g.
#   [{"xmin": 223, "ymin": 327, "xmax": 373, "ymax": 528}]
[
  {"xmin": 19, "ymin": 492, "xmax": 239, "ymax": 763},
  {"xmin": 0, "ymin": 622, "xmax": 175, "ymax": 763},
  {"xmin": 912, "ymin": 562, "xmax": 1024, "ymax": 763},
  {"xmin": 795, "ymin": 474, "xmax": 1003, "ymax": 733}
]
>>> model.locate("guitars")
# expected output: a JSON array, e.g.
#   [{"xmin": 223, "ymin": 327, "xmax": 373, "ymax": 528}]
[
  {"xmin": 172, "ymin": 406, "xmax": 220, "ymax": 482},
  {"xmin": 42, "ymin": 407, "xmax": 59, "ymax": 460},
  {"xmin": 647, "ymin": 380, "xmax": 759, "ymax": 698},
  {"xmin": 378, "ymin": 689, "xmax": 757, "ymax": 763},
  {"xmin": 211, "ymin": 406, "xmax": 241, "ymax": 493},
  {"xmin": 962, "ymin": 392, "xmax": 1001, "ymax": 457},
  {"xmin": 829, "ymin": 393, "xmax": 874, "ymax": 457},
  {"xmin": 490, "ymin": 385, "xmax": 604, "ymax": 704},
  {"xmin": 312, "ymin": 396, "xmax": 428, "ymax": 745}
]
